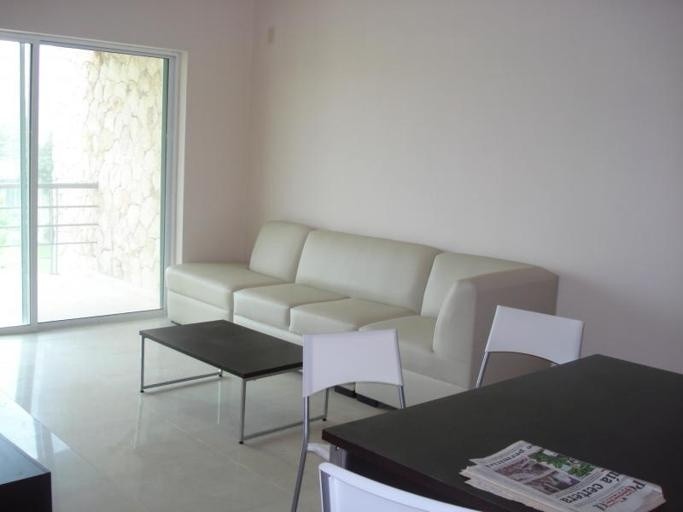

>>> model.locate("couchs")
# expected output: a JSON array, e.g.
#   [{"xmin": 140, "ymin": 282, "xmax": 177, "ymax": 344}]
[{"xmin": 165, "ymin": 220, "xmax": 559, "ymax": 409}]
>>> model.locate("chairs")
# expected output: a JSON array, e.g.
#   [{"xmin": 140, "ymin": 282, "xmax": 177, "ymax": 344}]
[
  {"xmin": 291, "ymin": 329, "xmax": 405, "ymax": 511},
  {"xmin": 318, "ymin": 461, "xmax": 486, "ymax": 511},
  {"xmin": 475, "ymin": 303, "xmax": 584, "ymax": 389}
]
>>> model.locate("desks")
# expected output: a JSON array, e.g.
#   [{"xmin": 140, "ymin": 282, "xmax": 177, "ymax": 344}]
[
  {"xmin": 322, "ymin": 353, "xmax": 683, "ymax": 511},
  {"xmin": 0, "ymin": 433, "xmax": 52, "ymax": 512}
]
